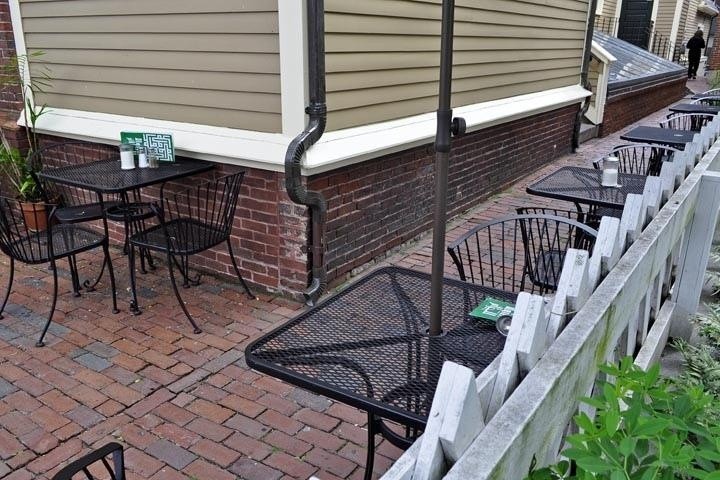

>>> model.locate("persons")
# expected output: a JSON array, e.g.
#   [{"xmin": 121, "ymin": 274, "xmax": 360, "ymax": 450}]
[{"xmin": 686, "ymin": 30, "xmax": 705, "ymax": 78}]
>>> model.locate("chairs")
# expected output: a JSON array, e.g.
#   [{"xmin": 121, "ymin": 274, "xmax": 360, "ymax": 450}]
[
  {"xmin": 0, "ymin": 196, "xmax": 121, "ymax": 348},
  {"xmin": 51, "ymin": 441, "xmax": 126, "ymax": 479},
  {"xmin": 612, "ymin": 144, "xmax": 652, "ymax": 157},
  {"xmin": 27, "ymin": 140, "xmax": 156, "ymax": 297},
  {"xmin": 446, "ymin": 87, "xmax": 720, "ymax": 369},
  {"xmin": 129, "ymin": 171, "xmax": 255, "ymax": 335}
]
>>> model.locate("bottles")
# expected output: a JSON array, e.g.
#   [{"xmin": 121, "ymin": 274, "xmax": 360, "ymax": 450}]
[
  {"xmin": 117, "ymin": 144, "xmax": 136, "ymax": 170},
  {"xmin": 139, "ymin": 147, "xmax": 148, "ymax": 168},
  {"xmin": 149, "ymin": 147, "xmax": 158, "ymax": 168},
  {"xmin": 602, "ymin": 155, "xmax": 619, "ymax": 187}
]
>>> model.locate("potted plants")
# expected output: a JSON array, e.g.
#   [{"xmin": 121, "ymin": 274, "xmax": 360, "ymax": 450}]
[{"xmin": 0, "ymin": 51, "xmax": 62, "ymax": 235}]
